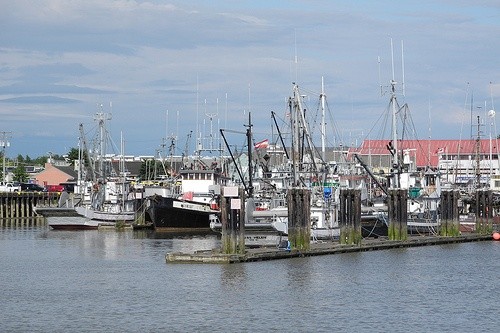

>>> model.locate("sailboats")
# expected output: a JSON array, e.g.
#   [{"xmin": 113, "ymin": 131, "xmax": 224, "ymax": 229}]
[{"xmin": 32, "ymin": 26, "xmax": 499, "ymax": 245}]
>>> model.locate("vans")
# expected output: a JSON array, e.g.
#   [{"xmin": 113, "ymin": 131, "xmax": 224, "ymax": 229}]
[{"xmin": 58, "ymin": 182, "xmax": 77, "ymax": 194}]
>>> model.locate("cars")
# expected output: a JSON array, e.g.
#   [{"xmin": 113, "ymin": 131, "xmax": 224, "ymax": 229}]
[{"xmin": 43, "ymin": 184, "xmax": 64, "ymax": 193}]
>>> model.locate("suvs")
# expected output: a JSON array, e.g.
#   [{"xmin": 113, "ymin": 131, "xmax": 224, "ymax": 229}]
[
  {"xmin": 19, "ymin": 183, "xmax": 44, "ymax": 193},
  {"xmin": 0, "ymin": 182, "xmax": 22, "ymax": 194}
]
